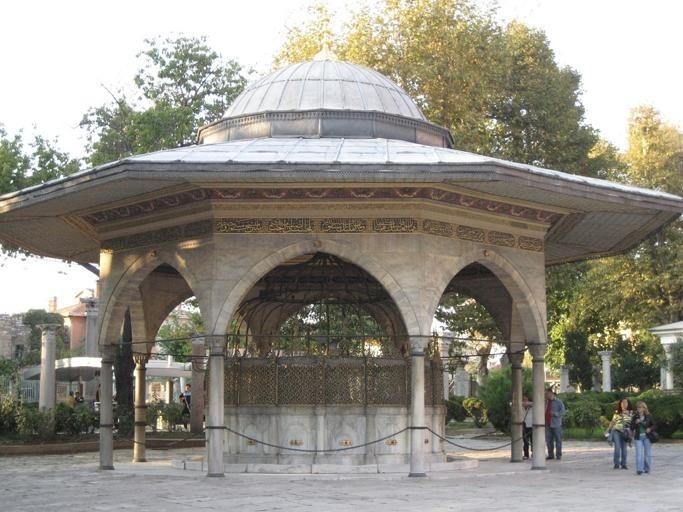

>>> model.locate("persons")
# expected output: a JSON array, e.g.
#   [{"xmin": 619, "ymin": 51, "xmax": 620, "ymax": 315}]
[
  {"xmin": 545, "ymin": 389, "xmax": 565, "ymax": 460},
  {"xmin": 179, "ymin": 384, "xmax": 191, "ymax": 429},
  {"xmin": 604, "ymin": 397, "xmax": 633, "ymax": 470},
  {"xmin": 66, "ymin": 390, "xmax": 77, "ymax": 409},
  {"xmin": 75, "ymin": 392, "xmax": 84, "ymax": 405},
  {"xmin": 630, "ymin": 399, "xmax": 656, "ymax": 475},
  {"xmin": 522, "ymin": 394, "xmax": 534, "ymax": 460}
]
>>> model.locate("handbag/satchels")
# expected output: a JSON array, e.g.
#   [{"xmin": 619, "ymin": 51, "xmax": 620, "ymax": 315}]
[
  {"xmin": 623, "ymin": 428, "xmax": 634, "ymax": 442},
  {"xmin": 646, "ymin": 430, "xmax": 659, "ymax": 443}
]
